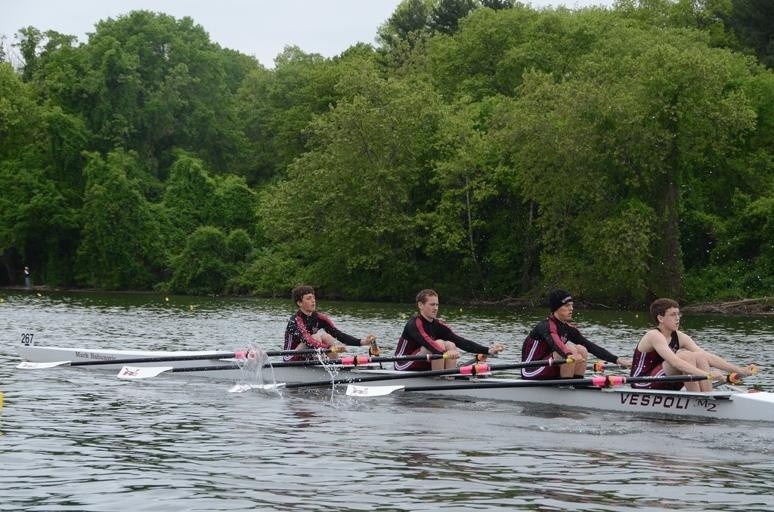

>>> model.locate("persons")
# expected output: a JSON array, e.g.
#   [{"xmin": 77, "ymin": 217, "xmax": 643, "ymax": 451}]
[
  {"xmin": 23, "ymin": 267, "xmax": 32, "ymax": 287},
  {"xmin": 394, "ymin": 289, "xmax": 497, "ymax": 374},
  {"xmin": 520, "ymin": 289, "xmax": 627, "ymax": 380},
  {"xmin": 282, "ymin": 286, "xmax": 373, "ymax": 360},
  {"xmin": 630, "ymin": 297, "xmax": 757, "ymax": 392}
]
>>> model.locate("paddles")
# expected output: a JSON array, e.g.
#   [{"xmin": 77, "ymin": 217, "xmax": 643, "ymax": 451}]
[
  {"xmin": 230, "ymin": 352, "xmax": 585, "ymax": 393},
  {"xmin": 347, "ymin": 373, "xmax": 725, "ymax": 397},
  {"xmin": 15, "ymin": 344, "xmax": 349, "ymax": 371},
  {"xmin": 116, "ymin": 353, "xmax": 460, "ymax": 380}
]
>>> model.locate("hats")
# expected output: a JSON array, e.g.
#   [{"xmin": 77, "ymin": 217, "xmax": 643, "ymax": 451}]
[{"xmin": 549, "ymin": 289, "xmax": 573, "ymax": 312}]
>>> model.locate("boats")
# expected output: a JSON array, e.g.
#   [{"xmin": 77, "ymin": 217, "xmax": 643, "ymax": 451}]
[{"xmin": 15, "ymin": 343, "xmax": 774, "ymax": 424}]
